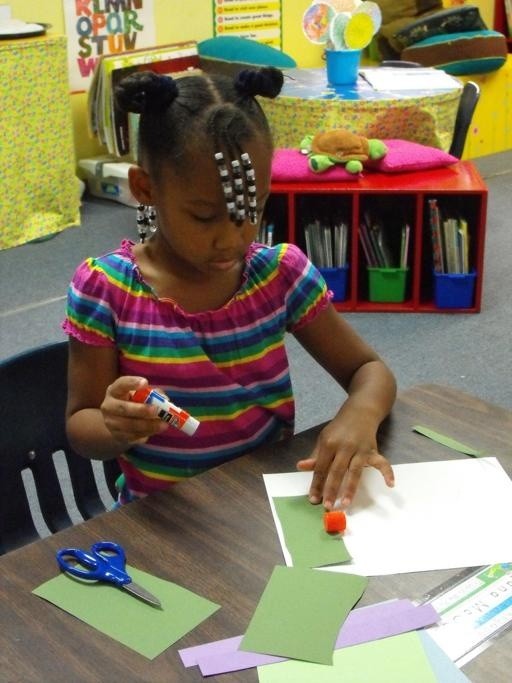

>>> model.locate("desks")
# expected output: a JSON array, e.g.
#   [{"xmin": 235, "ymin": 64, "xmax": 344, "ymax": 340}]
[
  {"xmin": 254, "ymin": 63, "xmax": 466, "ymax": 152},
  {"xmin": 1, "ymin": 379, "xmax": 512, "ymax": 683}
]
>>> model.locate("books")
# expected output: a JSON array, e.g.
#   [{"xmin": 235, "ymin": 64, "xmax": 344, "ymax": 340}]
[{"xmin": 246, "ymin": 192, "xmax": 479, "ymax": 280}]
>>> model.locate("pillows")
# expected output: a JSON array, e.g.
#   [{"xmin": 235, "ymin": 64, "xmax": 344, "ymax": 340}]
[
  {"xmin": 361, "ymin": 136, "xmax": 462, "ymax": 174},
  {"xmin": 369, "ymin": 0, "xmax": 508, "ymax": 73}
]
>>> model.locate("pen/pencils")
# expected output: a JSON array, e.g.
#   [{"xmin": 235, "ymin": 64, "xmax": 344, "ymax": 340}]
[{"xmin": 358, "ymin": 72, "xmax": 378, "ymax": 92}]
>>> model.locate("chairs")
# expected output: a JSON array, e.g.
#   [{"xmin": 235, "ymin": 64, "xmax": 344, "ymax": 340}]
[
  {"xmin": 447, "ymin": 77, "xmax": 482, "ymax": 161},
  {"xmin": 0, "ymin": 339, "xmax": 124, "ymax": 560}
]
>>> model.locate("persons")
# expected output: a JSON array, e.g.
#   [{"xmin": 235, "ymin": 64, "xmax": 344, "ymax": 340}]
[{"xmin": 59, "ymin": 62, "xmax": 400, "ymax": 519}]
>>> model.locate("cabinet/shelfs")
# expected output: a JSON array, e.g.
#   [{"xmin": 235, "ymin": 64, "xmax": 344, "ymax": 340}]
[{"xmin": 255, "ymin": 158, "xmax": 490, "ymax": 315}]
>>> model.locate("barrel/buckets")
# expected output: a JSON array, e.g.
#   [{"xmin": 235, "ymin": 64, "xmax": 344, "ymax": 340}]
[{"xmin": 320, "ymin": 47, "xmax": 362, "ymax": 86}]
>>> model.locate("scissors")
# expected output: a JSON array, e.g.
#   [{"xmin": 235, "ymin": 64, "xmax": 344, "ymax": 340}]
[{"xmin": 56, "ymin": 541, "xmax": 161, "ymax": 606}]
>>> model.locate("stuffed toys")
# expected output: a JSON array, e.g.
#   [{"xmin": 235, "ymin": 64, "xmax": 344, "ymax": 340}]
[{"xmin": 296, "ymin": 128, "xmax": 389, "ymax": 177}]
